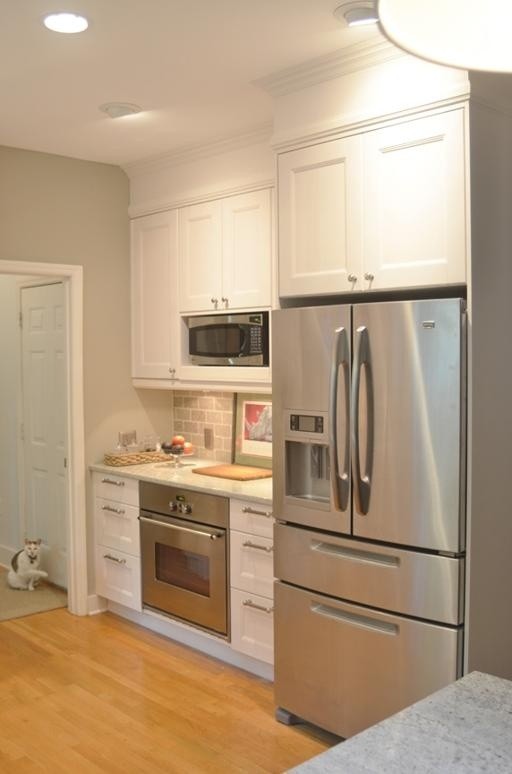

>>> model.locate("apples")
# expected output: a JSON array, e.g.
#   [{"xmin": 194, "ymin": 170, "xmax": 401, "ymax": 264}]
[{"xmin": 174, "ymin": 435, "xmax": 191, "ymax": 454}]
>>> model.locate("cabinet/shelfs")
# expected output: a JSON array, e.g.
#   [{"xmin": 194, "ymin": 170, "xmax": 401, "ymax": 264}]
[
  {"xmin": 227, "ymin": 489, "xmax": 275, "ymax": 675},
  {"xmin": 127, "ymin": 196, "xmax": 181, "ymax": 386},
  {"xmin": 180, "ymin": 179, "xmax": 275, "ymax": 316},
  {"xmin": 272, "ymin": 92, "xmax": 473, "ymax": 308},
  {"xmin": 86, "ymin": 464, "xmax": 142, "ymax": 620}
]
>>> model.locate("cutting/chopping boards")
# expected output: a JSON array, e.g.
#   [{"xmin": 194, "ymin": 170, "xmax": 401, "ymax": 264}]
[{"xmin": 191, "ymin": 462, "xmax": 272, "ymax": 482}]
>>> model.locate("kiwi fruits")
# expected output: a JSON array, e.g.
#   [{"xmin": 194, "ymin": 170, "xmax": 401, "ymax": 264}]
[{"xmin": 162, "ymin": 440, "xmax": 185, "ymax": 453}]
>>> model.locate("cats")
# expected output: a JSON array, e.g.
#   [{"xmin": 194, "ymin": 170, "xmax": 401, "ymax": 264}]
[{"xmin": 7, "ymin": 538, "xmax": 48, "ymax": 591}]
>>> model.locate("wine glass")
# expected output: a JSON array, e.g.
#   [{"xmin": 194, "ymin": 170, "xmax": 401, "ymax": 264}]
[{"xmin": 160, "ymin": 445, "xmax": 194, "ymax": 467}]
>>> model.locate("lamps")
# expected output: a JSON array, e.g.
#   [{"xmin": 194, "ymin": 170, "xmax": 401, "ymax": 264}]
[
  {"xmin": 331, "ymin": 0, "xmax": 382, "ymax": 30},
  {"xmin": 101, "ymin": 100, "xmax": 143, "ymax": 121}
]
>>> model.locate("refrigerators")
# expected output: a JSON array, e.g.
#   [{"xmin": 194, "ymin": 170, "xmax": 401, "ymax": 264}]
[{"xmin": 268, "ymin": 300, "xmax": 472, "ymax": 740}]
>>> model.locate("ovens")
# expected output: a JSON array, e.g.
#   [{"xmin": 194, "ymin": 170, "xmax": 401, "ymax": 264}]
[{"xmin": 136, "ymin": 479, "xmax": 231, "ymax": 643}]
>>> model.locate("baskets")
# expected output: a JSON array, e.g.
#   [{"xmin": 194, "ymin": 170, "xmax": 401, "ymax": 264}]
[{"xmin": 104, "ymin": 452, "xmax": 171, "ymax": 466}]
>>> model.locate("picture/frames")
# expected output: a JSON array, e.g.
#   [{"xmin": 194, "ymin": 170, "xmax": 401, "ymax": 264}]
[{"xmin": 230, "ymin": 392, "xmax": 272, "ymax": 468}]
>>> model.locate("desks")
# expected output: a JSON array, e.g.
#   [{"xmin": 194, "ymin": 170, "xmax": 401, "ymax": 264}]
[{"xmin": 277, "ymin": 668, "xmax": 511, "ymax": 773}]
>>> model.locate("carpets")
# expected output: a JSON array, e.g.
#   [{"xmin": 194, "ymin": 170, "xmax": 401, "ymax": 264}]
[{"xmin": 2, "ymin": 567, "xmax": 70, "ymax": 623}]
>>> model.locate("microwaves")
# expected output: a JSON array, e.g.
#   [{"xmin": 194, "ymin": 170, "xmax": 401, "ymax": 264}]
[{"xmin": 187, "ymin": 311, "xmax": 266, "ymax": 367}]
